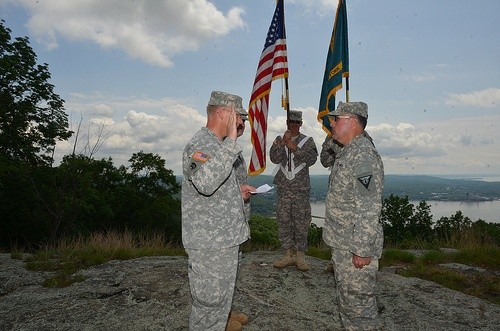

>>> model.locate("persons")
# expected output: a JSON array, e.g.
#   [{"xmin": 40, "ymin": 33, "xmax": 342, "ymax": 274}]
[
  {"xmin": 321, "ymin": 101, "xmax": 384, "ymax": 330},
  {"xmin": 270, "ymin": 111, "xmax": 318, "ymax": 271},
  {"xmin": 182, "ymin": 91, "xmax": 256, "ymax": 331}
]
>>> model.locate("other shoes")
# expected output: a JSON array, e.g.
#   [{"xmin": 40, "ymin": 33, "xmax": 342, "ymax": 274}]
[
  {"xmin": 225, "ymin": 319, "xmax": 242, "ymax": 331},
  {"xmin": 229, "ymin": 313, "xmax": 248, "ymax": 324}
]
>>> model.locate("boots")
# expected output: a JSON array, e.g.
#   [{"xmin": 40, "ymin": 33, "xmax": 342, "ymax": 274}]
[
  {"xmin": 295, "ymin": 251, "xmax": 309, "ymax": 270},
  {"xmin": 274, "ymin": 249, "xmax": 296, "ymax": 268}
]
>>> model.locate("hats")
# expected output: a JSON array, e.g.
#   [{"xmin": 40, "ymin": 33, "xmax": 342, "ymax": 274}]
[
  {"xmin": 242, "ymin": 116, "xmax": 248, "ymax": 120},
  {"xmin": 327, "ymin": 102, "xmax": 368, "ymax": 119},
  {"xmin": 208, "ymin": 91, "xmax": 249, "ymax": 115},
  {"xmin": 290, "ymin": 110, "xmax": 302, "ymax": 121}
]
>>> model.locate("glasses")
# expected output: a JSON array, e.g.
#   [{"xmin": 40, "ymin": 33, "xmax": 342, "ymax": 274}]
[
  {"xmin": 290, "ymin": 121, "xmax": 300, "ymax": 124},
  {"xmin": 334, "ymin": 118, "xmax": 350, "ymax": 122}
]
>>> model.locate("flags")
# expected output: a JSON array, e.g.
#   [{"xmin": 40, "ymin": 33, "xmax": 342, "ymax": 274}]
[
  {"xmin": 317, "ymin": 0, "xmax": 349, "ymax": 139},
  {"xmin": 247, "ymin": 0, "xmax": 289, "ymax": 176}
]
